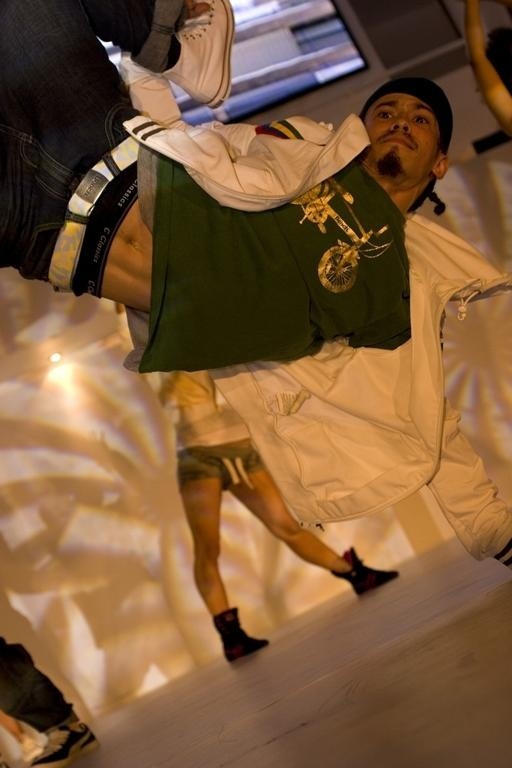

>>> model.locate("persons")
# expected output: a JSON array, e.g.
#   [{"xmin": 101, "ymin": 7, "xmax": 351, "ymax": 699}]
[
  {"xmin": 1, "ymin": 711, "xmax": 43, "ymax": 762},
  {"xmin": 144, "ymin": 368, "xmax": 400, "ymax": 665},
  {"xmin": 454, "ymin": 1, "xmax": 512, "ymax": 138},
  {"xmin": 3, "ymin": 1, "xmax": 511, "ymax": 576},
  {"xmin": 2, "ymin": 631, "xmax": 101, "ymax": 768}
]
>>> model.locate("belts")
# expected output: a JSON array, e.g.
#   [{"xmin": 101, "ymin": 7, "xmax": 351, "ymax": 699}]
[{"xmin": 47, "ymin": 136, "xmax": 140, "ymax": 293}]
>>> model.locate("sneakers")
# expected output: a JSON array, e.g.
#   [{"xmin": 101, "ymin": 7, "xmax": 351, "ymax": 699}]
[
  {"xmin": 163, "ymin": 0, "xmax": 235, "ymax": 108},
  {"xmin": 27, "ymin": 718, "xmax": 101, "ymax": 767}
]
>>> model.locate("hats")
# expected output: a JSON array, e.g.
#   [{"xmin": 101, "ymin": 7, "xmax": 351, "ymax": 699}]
[{"xmin": 356, "ymin": 76, "xmax": 452, "ymax": 153}]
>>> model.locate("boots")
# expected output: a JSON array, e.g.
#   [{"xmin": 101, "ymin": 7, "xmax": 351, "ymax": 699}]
[
  {"xmin": 331, "ymin": 546, "xmax": 399, "ymax": 595},
  {"xmin": 211, "ymin": 605, "xmax": 270, "ymax": 662}
]
